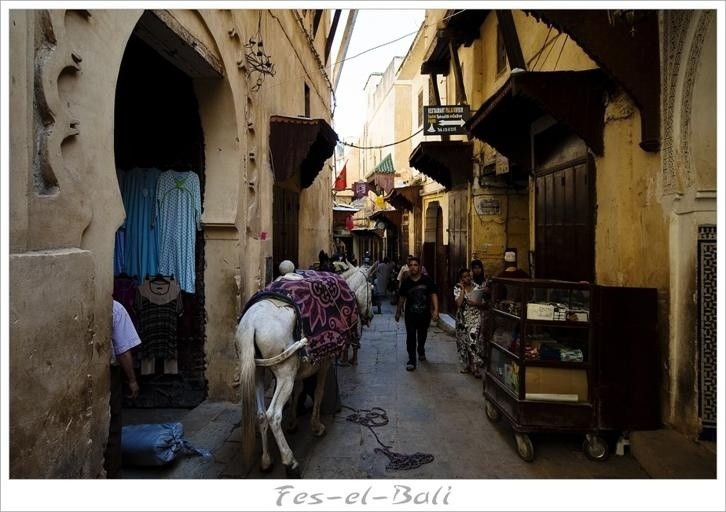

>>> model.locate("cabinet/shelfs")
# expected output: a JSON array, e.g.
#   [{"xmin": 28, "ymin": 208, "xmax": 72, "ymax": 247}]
[{"xmin": 483, "ymin": 278, "xmax": 663, "ymax": 433}]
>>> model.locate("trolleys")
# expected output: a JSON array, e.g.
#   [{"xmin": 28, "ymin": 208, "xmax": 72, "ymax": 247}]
[{"xmin": 484, "ymin": 278, "xmax": 610, "ymax": 462}]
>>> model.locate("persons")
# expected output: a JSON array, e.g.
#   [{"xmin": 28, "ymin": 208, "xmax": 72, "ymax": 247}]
[
  {"xmin": 341, "ymin": 253, "xmax": 494, "ymax": 378},
  {"xmin": 103, "ymin": 299, "xmax": 143, "ymax": 479}
]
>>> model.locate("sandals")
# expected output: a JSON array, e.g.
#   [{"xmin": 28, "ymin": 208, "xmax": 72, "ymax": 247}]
[
  {"xmin": 407, "ymin": 348, "xmax": 426, "ymax": 371},
  {"xmin": 461, "ymin": 366, "xmax": 482, "ymax": 378}
]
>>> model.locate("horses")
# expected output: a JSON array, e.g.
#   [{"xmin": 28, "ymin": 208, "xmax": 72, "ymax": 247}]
[{"xmin": 234, "ymin": 258, "xmax": 380, "ymax": 479}]
